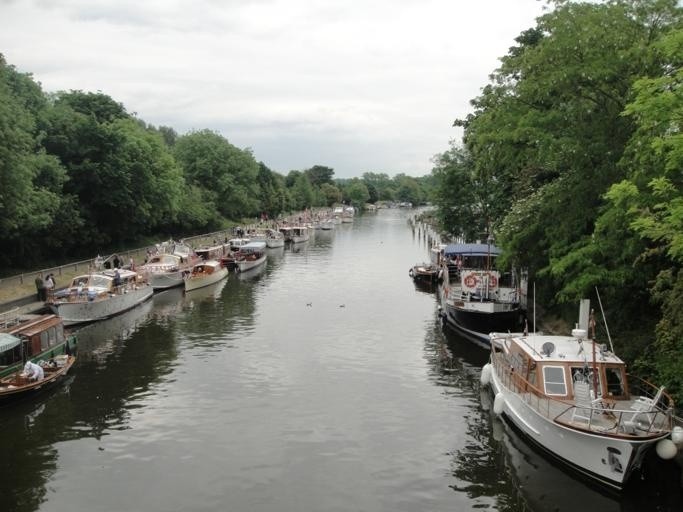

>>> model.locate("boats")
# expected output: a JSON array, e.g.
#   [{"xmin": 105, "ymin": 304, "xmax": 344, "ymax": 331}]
[
  {"xmin": 476, "ymin": 386, "xmax": 621, "ymax": 512},
  {"xmin": 408, "ymin": 261, "xmax": 440, "ymax": 288},
  {"xmin": 0, "ymin": 354, "xmax": 76, "ymax": 393},
  {"xmin": 61, "ymin": 297, "xmax": 156, "ymax": 352},
  {"xmin": 135, "ymin": 199, "xmax": 354, "ymax": 294},
  {"xmin": 361, "ymin": 201, "xmax": 415, "ymax": 213},
  {"xmin": 44, "ymin": 257, "xmax": 154, "ymax": 329},
  {"xmin": 474, "ymin": 293, "xmax": 681, "ymax": 505},
  {"xmin": 0, "ymin": 306, "xmax": 78, "ymax": 378},
  {"xmin": 1, "ymin": 374, "xmax": 75, "ymax": 424},
  {"xmin": 429, "ymin": 241, "xmax": 542, "ymax": 375}
]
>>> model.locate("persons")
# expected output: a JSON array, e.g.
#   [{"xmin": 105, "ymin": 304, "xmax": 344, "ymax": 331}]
[
  {"xmin": 34, "ymin": 272, "xmax": 46, "ymax": 302},
  {"xmin": 48, "ymin": 273, "xmax": 56, "ymax": 290},
  {"xmin": 103, "ymin": 257, "xmax": 110, "ymax": 269},
  {"xmin": 42, "ymin": 275, "xmax": 53, "ymax": 292},
  {"xmin": 113, "ymin": 256, "xmax": 120, "ymax": 268},
  {"xmin": 113, "ymin": 268, "xmax": 120, "ymax": 287},
  {"xmin": 22, "ymin": 360, "xmax": 43, "ymax": 382}
]
